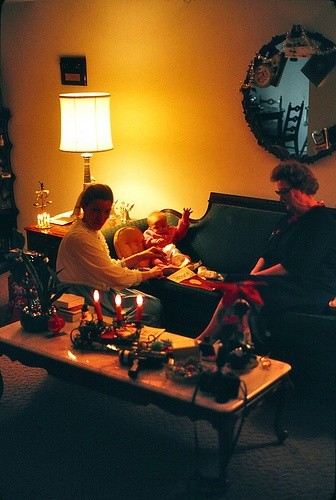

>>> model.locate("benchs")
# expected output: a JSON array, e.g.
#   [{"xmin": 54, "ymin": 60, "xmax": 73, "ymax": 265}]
[{"xmin": 102, "ymin": 191, "xmax": 336, "ymax": 370}]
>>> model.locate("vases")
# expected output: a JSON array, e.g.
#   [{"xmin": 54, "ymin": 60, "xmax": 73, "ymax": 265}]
[{"xmin": 16, "ymin": 304, "xmax": 56, "ymax": 331}]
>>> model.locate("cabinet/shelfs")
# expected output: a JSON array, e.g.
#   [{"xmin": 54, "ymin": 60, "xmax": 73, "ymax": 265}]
[{"xmin": 0, "ymin": 107, "xmax": 25, "ymax": 274}]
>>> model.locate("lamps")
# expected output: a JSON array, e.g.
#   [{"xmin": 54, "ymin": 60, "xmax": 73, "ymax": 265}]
[{"xmin": 57, "ymin": 93, "xmax": 115, "ymax": 191}]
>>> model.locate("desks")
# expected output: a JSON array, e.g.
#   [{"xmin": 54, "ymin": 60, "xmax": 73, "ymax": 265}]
[
  {"xmin": 25, "ymin": 217, "xmax": 139, "ymax": 281},
  {"xmin": 1, "ymin": 313, "xmax": 293, "ymax": 488}
]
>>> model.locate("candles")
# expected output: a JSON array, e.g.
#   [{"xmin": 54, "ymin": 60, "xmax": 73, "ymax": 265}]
[
  {"xmin": 137, "ymin": 295, "xmax": 145, "ymax": 324},
  {"xmin": 92, "ymin": 289, "xmax": 103, "ymax": 321},
  {"xmin": 115, "ymin": 294, "xmax": 126, "ymax": 323}
]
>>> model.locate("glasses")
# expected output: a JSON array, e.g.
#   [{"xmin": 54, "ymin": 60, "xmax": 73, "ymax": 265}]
[{"xmin": 275, "ymin": 186, "xmax": 292, "ymax": 196}]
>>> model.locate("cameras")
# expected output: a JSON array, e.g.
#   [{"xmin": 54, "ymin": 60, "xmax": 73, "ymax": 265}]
[{"xmin": 199, "ymin": 371, "xmax": 239, "ymax": 403}]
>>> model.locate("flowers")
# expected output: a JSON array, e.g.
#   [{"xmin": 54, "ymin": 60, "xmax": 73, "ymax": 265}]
[{"xmin": 7, "ymin": 252, "xmax": 69, "ymax": 315}]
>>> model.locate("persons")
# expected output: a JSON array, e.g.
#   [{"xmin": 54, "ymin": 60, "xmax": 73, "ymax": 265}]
[
  {"xmin": 195, "ymin": 161, "xmax": 336, "ymax": 345},
  {"xmin": 142, "ymin": 207, "xmax": 195, "ymax": 271},
  {"xmin": 55, "ymin": 184, "xmax": 182, "ymax": 328}
]
